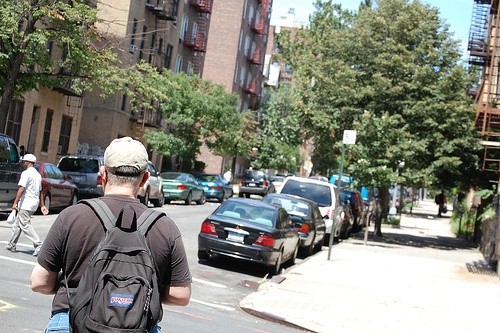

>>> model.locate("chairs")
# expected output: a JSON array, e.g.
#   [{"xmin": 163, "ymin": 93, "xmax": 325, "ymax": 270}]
[
  {"xmin": 234, "ymin": 208, "xmax": 252, "ymax": 219},
  {"xmin": 223, "ymin": 210, "xmax": 241, "ymax": 219},
  {"xmin": 284, "ymin": 184, "xmax": 303, "ymax": 197},
  {"xmin": 272, "ymin": 203, "xmax": 282, "ymax": 208},
  {"xmin": 313, "ymin": 193, "xmax": 329, "ymax": 204},
  {"xmin": 255, "ymin": 217, "xmax": 274, "ymax": 227},
  {"xmin": 299, "ymin": 207, "xmax": 308, "ymax": 215}
]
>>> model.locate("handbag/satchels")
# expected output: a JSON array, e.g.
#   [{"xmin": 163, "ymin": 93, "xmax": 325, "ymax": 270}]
[
  {"xmin": 7, "ymin": 209, "xmax": 16, "ymax": 224},
  {"xmin": 442, "ymin": 206, "xmax": 448, "ymax": 213}
]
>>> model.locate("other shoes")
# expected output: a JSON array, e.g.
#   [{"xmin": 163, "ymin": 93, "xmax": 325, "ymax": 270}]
[
  {"xmin": 6, "ymin": 245, "xmax": 16, "ymax": 252},
  {"xmin": 33, "ymin": 246, "xmax": 41, "ymax": 256}
]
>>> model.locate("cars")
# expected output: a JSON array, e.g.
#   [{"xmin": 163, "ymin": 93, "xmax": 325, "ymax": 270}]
[
  {"xmin": 260, "ymin": 192, "xmax": 329, "ymax": 259},
  {"xmin": 269, "ymin": 171, "xmax": 401, "ymax": 247},
  {"xmin": 34, "ymin": 161, "xmax": 79, "ymax": 214},
  {"xmin": 197, "ymin": 197, "xmax": 302, "ymax": 277},
  {"xmin": 158, "ymin": 171, "xmax": 206, "ymax": 206},
  {"xmin": 194, "ymin": 173, "xmax": 234, "ymax": 203}
]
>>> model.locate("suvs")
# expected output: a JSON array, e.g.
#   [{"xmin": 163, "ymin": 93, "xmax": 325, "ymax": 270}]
[
  {"xmin": 137, "ymin": 160, "xmax": 165, "ymax": 208},
  {"xmin": 57, "ymin": 154, "xmax": 107, "ymax": 198},
  {"xmin": 238, "ymin": 171, "xmax": 276, "ymax": 198}
]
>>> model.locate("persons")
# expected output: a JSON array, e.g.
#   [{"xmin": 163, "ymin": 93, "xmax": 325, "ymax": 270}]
[
  {"xmin": 20, "ymin": 145, "xmax": 29, "ymax": 157},
  {"xmin": 223, "ymin": 168, "xmax": 233, "ymax": 182},
  {"xmin": 5, "ymin": 153, "xmax": 48, "ymax": 256},
  {"xmin": 30, "ymin": 136, "xmax": 192, "ymax": 333},
  {"xmin": 437, "ymin": 190, "xmax": 447, "ymax": 217}
]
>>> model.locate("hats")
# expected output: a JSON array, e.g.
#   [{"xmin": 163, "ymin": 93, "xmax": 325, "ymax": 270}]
[
  {"xmin": 103, "ymin": 136, "xmax": 149, "ymax": 175},
  {"xmin": 19, "ymin": 154, "xmax": 37, "ymax": 163}
]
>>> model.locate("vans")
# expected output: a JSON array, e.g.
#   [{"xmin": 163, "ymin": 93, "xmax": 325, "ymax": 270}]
[{"xmin": 0, "ymin": 133, "xmax": 27, "ymax": 218}]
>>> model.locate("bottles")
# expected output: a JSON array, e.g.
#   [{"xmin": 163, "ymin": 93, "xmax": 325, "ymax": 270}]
[
  {"xmin": 41, "ymin": 206, "xmax": 49, "ymax": 216},
  {"xmin": 7, "ymin": 207, "xmax": 16, "ymax": 224}
]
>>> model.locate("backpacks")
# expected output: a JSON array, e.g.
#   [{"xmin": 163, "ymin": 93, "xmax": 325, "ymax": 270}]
[
  {"xmin": 435, "ymin": 194, "xmax": 441, "ymax": 204},
  {"xmin": 61, "ymin": 198, "xmax": 168, "ymax": 333}
]
